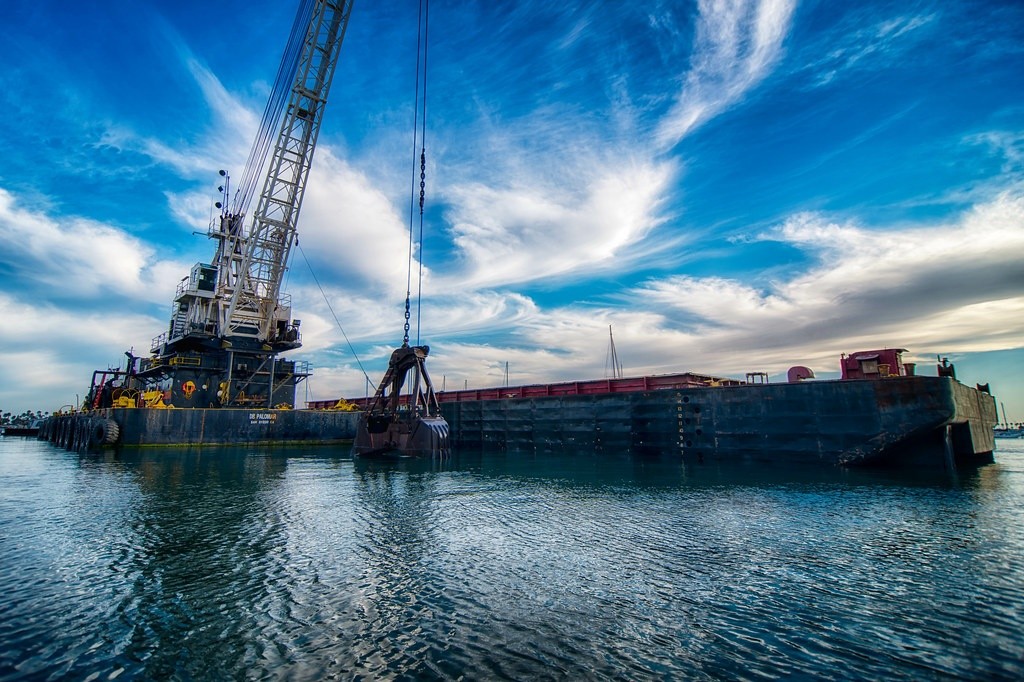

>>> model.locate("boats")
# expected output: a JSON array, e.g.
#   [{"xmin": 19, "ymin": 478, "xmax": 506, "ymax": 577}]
[{"xmin": 36, "ymin": 402, "xmax": 430, "ymax": 458}]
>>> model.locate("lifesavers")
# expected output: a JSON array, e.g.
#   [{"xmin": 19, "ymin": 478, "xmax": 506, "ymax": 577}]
[
  {"xmin": 92, "ymin": 418, "xmax": 119, "ymax": 447},
  {"xmin": 39, "ymin": 417, "xmax": 98, "ymax": 452},
  {"xmin": 183, "ymin": 382, "xmax": 196, "ymax": 393}
]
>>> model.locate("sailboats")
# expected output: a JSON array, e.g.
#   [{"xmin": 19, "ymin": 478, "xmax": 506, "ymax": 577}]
[{"xmin": 994, "ymin": 401, "xmax": 1023, "ymax": 439}]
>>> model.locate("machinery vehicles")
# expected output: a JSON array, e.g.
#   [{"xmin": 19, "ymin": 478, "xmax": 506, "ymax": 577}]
[{"xmin": 121, "ymin": 0, "xmax": 452, "ymax": 454}]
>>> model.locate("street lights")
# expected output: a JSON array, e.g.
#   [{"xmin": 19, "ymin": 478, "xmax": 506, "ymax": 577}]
[
  {"xmin": 123, "ymin": 350, "xmax": 134, "ymax": 399},
  {"xmin": 75, "ymin": 393, "xmax": 79, "ymax": 411}
]
